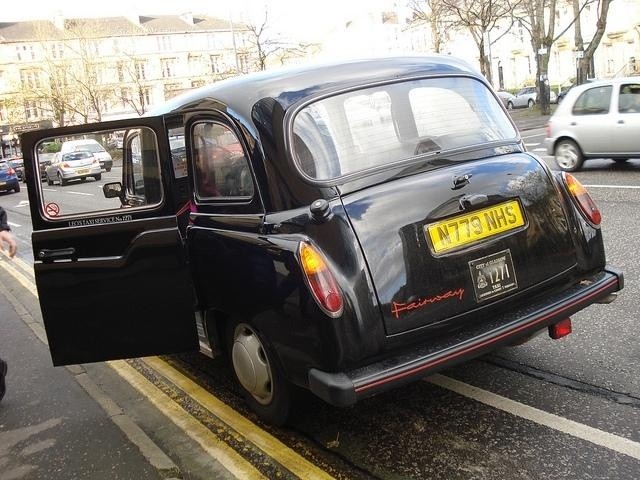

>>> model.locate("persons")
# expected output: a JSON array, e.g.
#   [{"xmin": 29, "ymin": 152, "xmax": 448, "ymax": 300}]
[{"xmin": 0, "ymin": 205, "xmax": 18, "ymax": 404}]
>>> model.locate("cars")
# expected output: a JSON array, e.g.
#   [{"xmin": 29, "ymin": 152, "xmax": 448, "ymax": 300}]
[
  {"xmin": 545, "ymin": 76, "xmax": 640, "ymax": 173},
  {"xmin": 495, "ymin": 91, "xmax": 515, "ymax": 109},
  {"xmin": 0, "ymin": 128, "xmax": 248, "ymax": 197},
  {"xmin": 19, "ymin": 53, "xmax": 624, "ymax": 428},
  {"xmin": 507, "ymin": 86, "xmax": 557, "ymax": 111},
  {"xmin": 556, "ymin": 78, "xmax": 600, "ymax": 105}
]
